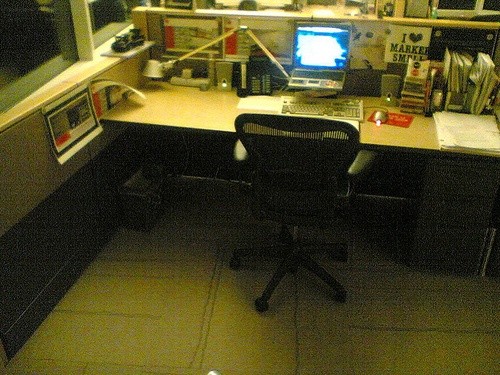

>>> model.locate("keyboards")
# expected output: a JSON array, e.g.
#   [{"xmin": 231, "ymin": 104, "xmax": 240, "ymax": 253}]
[{"xmin": 281, "ymin": 97, "xmax": 364, "ymax": 120}]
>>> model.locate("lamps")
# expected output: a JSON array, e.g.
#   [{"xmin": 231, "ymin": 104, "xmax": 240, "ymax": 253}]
[{"xmin": 142, "ymin": 25, "xmax": 290, "ymax": 111}]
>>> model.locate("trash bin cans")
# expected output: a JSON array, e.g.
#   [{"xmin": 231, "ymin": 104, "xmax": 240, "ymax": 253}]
[{"xmin": 106, "ymin": 167, "xmax": 170, "ymax": 232}]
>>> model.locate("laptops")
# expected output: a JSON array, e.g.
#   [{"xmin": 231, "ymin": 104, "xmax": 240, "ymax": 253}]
[{"xmin": 286, "ymin": 20, "xmax": 352, "ymax": 91}]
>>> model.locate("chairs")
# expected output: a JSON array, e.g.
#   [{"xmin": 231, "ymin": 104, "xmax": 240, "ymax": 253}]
[{"xmin": 230, "ymin": 113, "xmax": 360, "ymax": 313}]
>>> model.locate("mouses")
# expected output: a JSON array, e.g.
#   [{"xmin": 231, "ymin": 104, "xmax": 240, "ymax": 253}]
[{"xmin": 374, "ymin": 110, "xmax": 389, "ymax": 123}]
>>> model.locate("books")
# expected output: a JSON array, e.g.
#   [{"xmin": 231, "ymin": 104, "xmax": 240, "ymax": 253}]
[{"xmin": 443, "ymin": 48, "xmax": 500, "ymax": 114}]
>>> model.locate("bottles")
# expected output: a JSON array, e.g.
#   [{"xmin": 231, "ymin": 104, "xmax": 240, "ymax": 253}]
[
  {"xmin": 235, "ymin": 61, "xmax": 252, "ymax": 97},
  {"xmin": 430, "ymin": 7, "xmax": 438, "ymax": 19}
]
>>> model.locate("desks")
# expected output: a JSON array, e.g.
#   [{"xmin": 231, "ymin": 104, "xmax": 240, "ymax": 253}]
[{"xmin": 99, "ymin": 80, "xmax": 500, "ymax": 277}]
